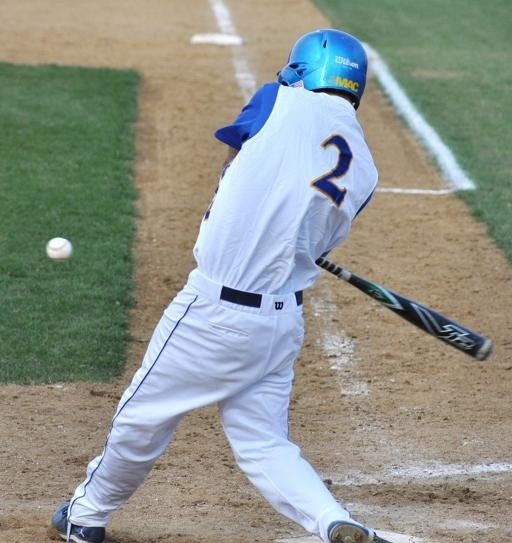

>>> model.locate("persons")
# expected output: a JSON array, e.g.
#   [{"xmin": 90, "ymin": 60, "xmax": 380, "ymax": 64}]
[{"xmin": 47, "ymin": 28, "xmax": 399, "ymax": 543}]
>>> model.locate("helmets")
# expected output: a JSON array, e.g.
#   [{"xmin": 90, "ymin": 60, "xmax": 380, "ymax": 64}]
[{"xmin": 278, "ymin": 30, "xmax": 368, "ymax": 109}]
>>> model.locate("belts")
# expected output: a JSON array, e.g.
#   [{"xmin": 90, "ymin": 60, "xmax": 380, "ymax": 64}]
[{"xmin": 220, "ymin": 286, "xmax": 304, "ymax": 308}]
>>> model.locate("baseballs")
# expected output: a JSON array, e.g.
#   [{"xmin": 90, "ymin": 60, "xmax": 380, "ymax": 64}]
[{"xmin": 45, "ymin": 237, "xmax": 73, "ymax": 261}]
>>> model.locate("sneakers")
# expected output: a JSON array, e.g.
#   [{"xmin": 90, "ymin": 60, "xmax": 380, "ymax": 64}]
[
  {"xmin": 327, "ymin": 520, "xmax": 393, "ymax": 543},
  {"xmin": 52, "ymin": 501, "xmax": 105, "ymax": 543}
]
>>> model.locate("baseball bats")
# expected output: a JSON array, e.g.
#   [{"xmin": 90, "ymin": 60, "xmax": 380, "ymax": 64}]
[{"xmin": 316, "ymin": 257, "xmax": 492, "ymax": 362}]
